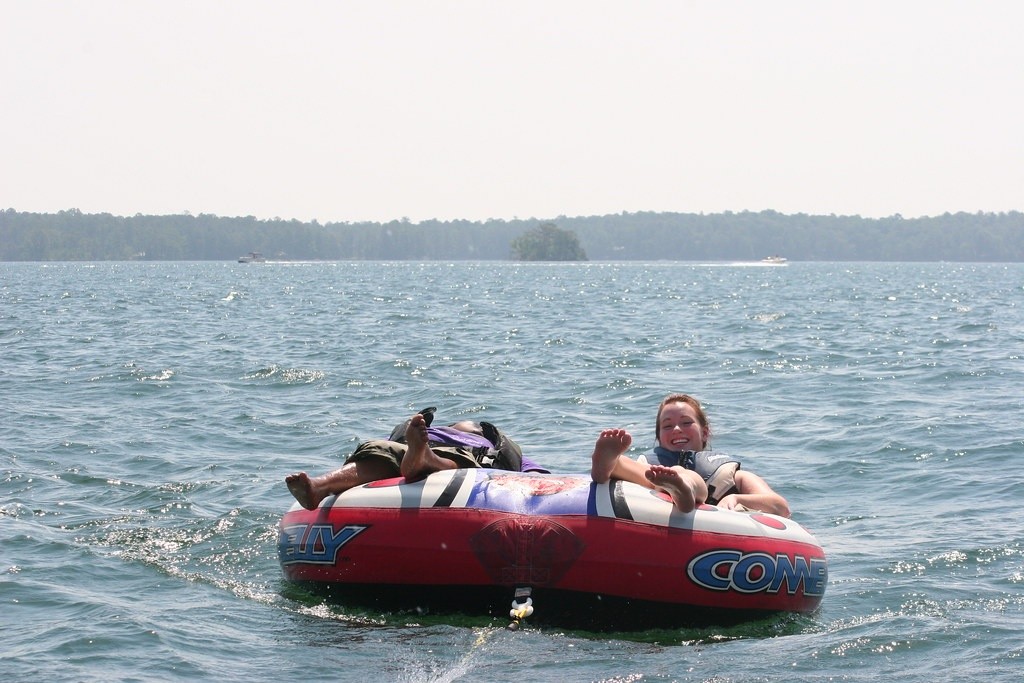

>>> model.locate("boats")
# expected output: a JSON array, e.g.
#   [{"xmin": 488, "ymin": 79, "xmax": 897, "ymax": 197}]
[{"xmin": 276, "ymin": 467, "xmax": 831, "ymax": 622}]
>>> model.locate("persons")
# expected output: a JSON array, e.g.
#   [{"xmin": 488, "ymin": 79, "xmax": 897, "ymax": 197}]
[
  {"xmin": 285, "ymin": 407, "xmax": 550, "ymax": 512},
  {"xmin": 592, "ymin": 395, "xmax": 791, "ymax": 519}
]
